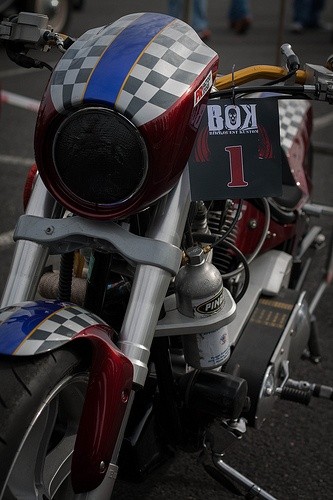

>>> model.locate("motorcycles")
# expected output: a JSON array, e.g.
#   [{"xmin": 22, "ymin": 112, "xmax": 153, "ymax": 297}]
[{"xmin": 0, "ymin": 0, "xmax": 333, "ymax": 500}]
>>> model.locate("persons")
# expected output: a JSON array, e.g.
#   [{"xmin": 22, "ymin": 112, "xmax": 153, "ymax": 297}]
[
  {"xmin": 290, "ymin": 1, "xmax": 333, "ymax": 36},
  {"xmin": 228, "ymin": 1, "xmax": 254, "ymax": 36},
  {"xmin": 166, "ymin": 1, "xmax": 212, "ymax": 43}
]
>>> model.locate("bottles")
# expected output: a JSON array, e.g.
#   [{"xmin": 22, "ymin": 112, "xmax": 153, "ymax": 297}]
[{"xmin": 175, "ymin": 246, "xmax": 231, "ymax": 371}]
